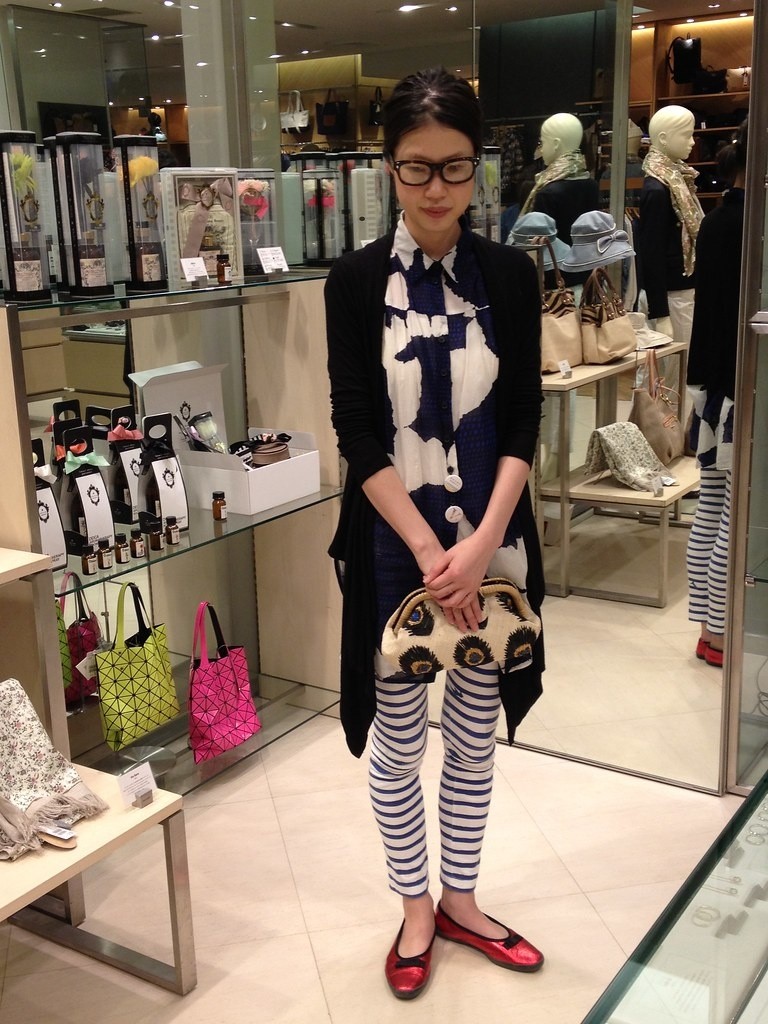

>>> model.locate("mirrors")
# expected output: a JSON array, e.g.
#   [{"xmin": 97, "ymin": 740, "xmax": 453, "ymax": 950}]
[{"xmin": 237, "ymin": 0, "xmax": 757, "ymax": 796}]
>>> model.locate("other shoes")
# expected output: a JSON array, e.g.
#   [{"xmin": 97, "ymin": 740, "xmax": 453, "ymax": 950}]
[
  {"xmin": 385, "ymin": 897, "xmax": 544, "ymax": 999},
  {"xmin": 695, "ymin": 639, "xmax": 723, "ymax": 667}
]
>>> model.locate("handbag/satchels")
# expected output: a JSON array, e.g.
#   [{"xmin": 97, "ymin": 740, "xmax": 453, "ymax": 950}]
[
  {"xmin": 381, "ymin": 575, "xmax": 541, "ymax": 674},
  {"xmin": 579, "ymin": 265, "xmax": 637, "ymax": 365},
  {"xmin": 725, "ymin": 66, "xmax": 752, "ymax": 92},
  {"xmin": 628, "ymin": 349, "xmax": 685, "ymax": 466},
  {"xmin": 529, "ymin": 236, "xmax": 583, "ymax": 374},
  {"xmin": 280, "ymin": 89, "xmax": 312, "ymax": 133},
  {"xmin": 189, "ymin": 601, "xmax": 264, "ymax": 765},
  {"xmin": 693, "ymin": 68, "xmax": 727, "ymax": 95},
  {"xmin": 368, "ymin": 87, "xmax": 385, "ymax": 127},
  {"xmin": 316, "ymin": 87, "xmax": 347, "ymax": 136},
  {"xmin": 55, "ymin": 571, "xmax": 104, "ymax": 710},
  {"xmin": 95, "ymin": 581, "xmax": 181, "ymax": 752}
]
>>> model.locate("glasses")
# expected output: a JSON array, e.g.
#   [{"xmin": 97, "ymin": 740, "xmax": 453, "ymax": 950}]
[{"xmin": 389, "ymin": 153, "xmax": 482, "ymax": 186}]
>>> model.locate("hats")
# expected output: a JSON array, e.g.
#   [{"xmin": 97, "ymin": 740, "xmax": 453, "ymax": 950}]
[
  {"xmin": 559, "ymin": 209, "xmax": 637, "ymax": 272},
  {"xmin": 628, "ymin": 310, "xmax": 674, "ymax": 349},
  {"xmin": 510, "ymin": 211, "xmax": 571, "ymax": 272}
]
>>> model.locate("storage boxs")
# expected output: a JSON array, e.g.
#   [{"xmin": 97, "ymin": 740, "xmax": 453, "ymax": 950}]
[{"xmin": 127, "ymin": 362, "xmax": 322, "ymax": 515}]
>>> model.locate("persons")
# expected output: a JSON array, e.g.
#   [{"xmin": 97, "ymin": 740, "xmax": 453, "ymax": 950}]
[
  {"xmin": 144, "ymin": 112, "xmax": 168, "ymax": 141},
  {"xmin": 500, "ymin": 192, "xmax": 521, "ymax": 245},
  {"xmin": 327, "ymin": 70, "xmax": 547, "ymax": 1000},
  {"xmin": 599, "ymin": 119, "xmax": 647, "ymax": 276},
  {"xmin": 509, "ymin": 112, "xmax": 596, "ymax": 486},
  {"xmin": 631, "ymin": 105, "xmax": 704, "ymax": 500},
  {"xmin": 682, "ymin": 118, "xmax": 748, "ymax": 668}
]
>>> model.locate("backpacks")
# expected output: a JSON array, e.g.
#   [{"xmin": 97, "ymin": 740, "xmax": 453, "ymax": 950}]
[{"xmin": 667, "ymin": 31, "xmax": 703, "ymax": 83}]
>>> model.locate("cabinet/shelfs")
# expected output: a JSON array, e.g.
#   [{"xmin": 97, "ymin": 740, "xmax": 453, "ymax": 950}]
[
  {"xmin": 630, "ymin": 12, "xmax": 753, "ymax": 197},
  {"xmin": 1, "ymin": 267, "xmax": 342, "ymax": 798},
  {"xmin": 537, "ymin": 262, "xmax": 701, "ymax": 605},
  {"xmin": 0, "ymin": 550, "xmax": 197, "ymax": 998}
]
